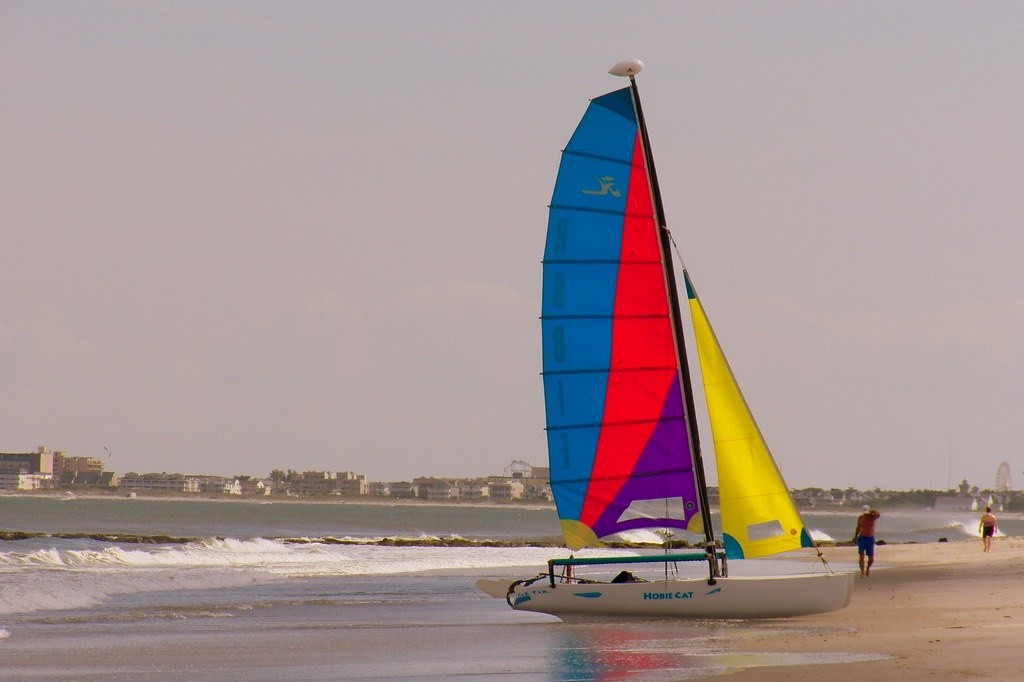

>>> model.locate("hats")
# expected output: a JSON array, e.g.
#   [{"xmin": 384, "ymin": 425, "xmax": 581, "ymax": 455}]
[{"xmin": 863, "ymin": 505, "xmax": 870, "ymax": 514}]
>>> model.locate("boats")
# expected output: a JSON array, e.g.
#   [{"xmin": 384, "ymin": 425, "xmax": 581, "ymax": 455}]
[
  {"xmin": 654, "ymin": 530, "xmax": 673, "ymax": 538},
  {"xmin": 809, "ymin": 530, "xmax": 836, "ymax": 546}
]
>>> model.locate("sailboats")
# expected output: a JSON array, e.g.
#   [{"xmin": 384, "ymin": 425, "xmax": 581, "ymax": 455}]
[{"xmin": 506, "ymin": 59, "xmax": 853, "ymax": 624}]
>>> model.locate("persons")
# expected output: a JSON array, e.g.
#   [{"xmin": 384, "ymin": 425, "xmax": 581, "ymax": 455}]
[
  {"xmin": 852, "ymin": 504, "xmax": 880, "ymax": 578},
  {"xmin": 979, "ymin": 506, "xmax": 997, "ymax": 553}
]
